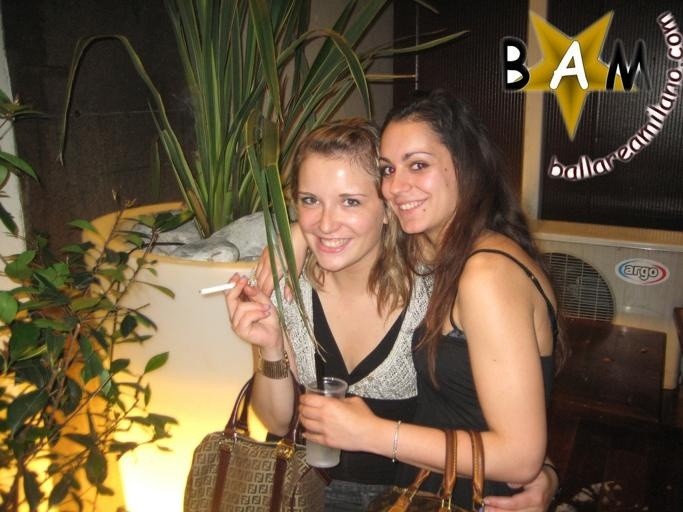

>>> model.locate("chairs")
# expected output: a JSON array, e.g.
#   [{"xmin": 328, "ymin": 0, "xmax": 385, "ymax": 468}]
[{"xmin": 546, "ymin": 316, "xmax": 666, "ymax": 512}]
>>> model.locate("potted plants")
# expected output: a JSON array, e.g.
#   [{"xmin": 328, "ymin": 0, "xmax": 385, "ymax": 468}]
[{"xmin": 53, "ymin": 0, "xmax": 467, "ymax": 435}]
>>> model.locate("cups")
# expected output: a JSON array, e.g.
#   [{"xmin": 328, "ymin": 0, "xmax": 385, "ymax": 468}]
[{"xmin": 304, "ymin": 376, "xmax": 346, "ymax": 469}]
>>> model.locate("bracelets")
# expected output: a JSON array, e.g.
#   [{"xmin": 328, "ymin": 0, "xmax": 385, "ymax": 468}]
[
  {"xmin": 257, "ymin": 350, "xmax": 290, "ymax": 380},
  {"xmin": 543, "ymin": 462, "xmax": 559, "ymax": 476},
  {"xmin": 392, "ymin": 420, "xmax": 402, "ymax": 465}
]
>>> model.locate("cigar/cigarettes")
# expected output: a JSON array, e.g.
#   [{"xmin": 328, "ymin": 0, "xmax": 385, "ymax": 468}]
[{"xmin": 198, "ymin": 280, "xmax": 250, "ymax": 296}]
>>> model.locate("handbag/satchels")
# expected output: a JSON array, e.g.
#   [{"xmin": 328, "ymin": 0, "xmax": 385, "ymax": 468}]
[
  {"xmin": 183, "ymin": 367, "xmax": 324, "ymax": 512},
  {"xmin": 324, "ymin": 428, "xmax": 488, "ymax": 512}
]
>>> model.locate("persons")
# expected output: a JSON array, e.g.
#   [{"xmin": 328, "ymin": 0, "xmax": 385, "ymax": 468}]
[
  {"xmin": 224, "ymin": 118, "xmax": 560, "ymax": 512},
  {"xmin": 298, "ymin": 85, "xmax": 560, "ymax": 512}
]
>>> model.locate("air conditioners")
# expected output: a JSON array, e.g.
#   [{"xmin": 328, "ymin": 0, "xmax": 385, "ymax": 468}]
[{"xmin": 521, "ymin": 232, "xmax": 683, "ymax": 390}]
